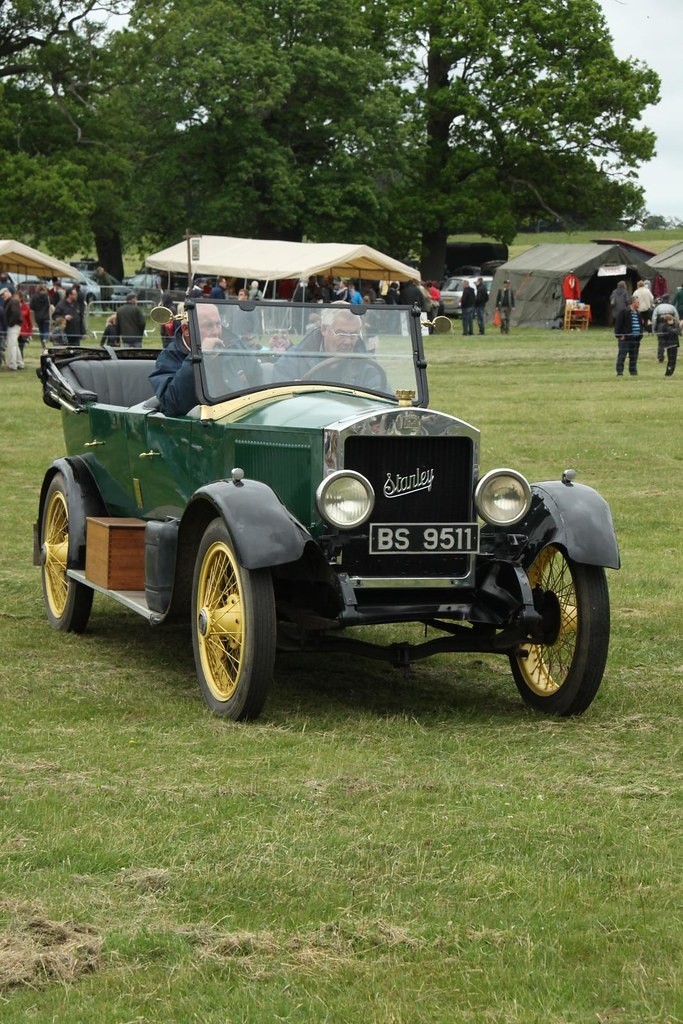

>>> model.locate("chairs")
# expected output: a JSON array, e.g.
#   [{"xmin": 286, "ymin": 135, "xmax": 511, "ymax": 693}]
[{"xmin": 71, "ymin": 360, "xmax": 293, "ymax": 414}]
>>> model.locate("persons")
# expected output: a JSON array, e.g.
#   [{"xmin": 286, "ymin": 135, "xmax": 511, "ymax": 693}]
[
  {"xmin": 271, "ymin": 300, "xmax": 395, "ymax": 398},
  {"xmin": 0, "ymin": 273, "xmax": 88, "ymax": 371},
  {"xmin": 96, "ymin": 267, "xmax": 114, "ymax": 311},
  {"xmin": 148, "ymin": 303, "xmax": 264, "ymax": 416},
  {"xmin": 100, "ymin": 294, "xmax": 146, "ymax": 348},
  {"xmin": 293, "ymin": 275, "xmax": 441, "ymax": 335},
  {"xmin": 461, "ymin": 277, "xmax": 489, "ymax": 336},
  {"xmin": 610, "ymin": 280, "xmax": 683, "ymax": 377},
  {"xmin": 495, "ymin": 280, "xmax": 515, "ymax": 335},
  {"xmin": 160, "ymin": 277, "xmax": 291, "ymax": 363}
]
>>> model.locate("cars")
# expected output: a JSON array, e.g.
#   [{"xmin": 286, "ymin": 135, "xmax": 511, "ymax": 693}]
[
  {"xmin": 5, "ymin": 272, "xmax": 55, "ymax": 306},
  {"xmin": 439, "ymin": 275, "xmax": 494, "ymax": 319},
  {"xmin": 481, "ymin": 260, "xmax": 507, "ymax": 275},
  {"xmin": 126, "ymin": 274, "xmax": 163, "ymax": 303},
  {"xmin": 59, "ymin": 260, "xmax": 100, "ymax": 272},
  {"xmin": 60, "ymin": 270, "xmax": 136, "ymax": 308},
  {"xmin": 30, "ymin": 228, "xmax": 622, "ymax": 725}
]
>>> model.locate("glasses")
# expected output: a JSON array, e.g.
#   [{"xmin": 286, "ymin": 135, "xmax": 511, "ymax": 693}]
[{"xmin": 331, "ymin": 329, "xmax": 362, "ymax": 339}]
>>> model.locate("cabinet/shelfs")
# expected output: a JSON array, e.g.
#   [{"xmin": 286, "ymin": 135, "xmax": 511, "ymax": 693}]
[{"xmin": 564, "ymin": 303, "xmax": 590, "ymax": 330}]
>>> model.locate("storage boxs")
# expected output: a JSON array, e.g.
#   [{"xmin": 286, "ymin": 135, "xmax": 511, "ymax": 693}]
[{"xmin": 85, "ymin": 516, "xmax": 149, "ymax": 590}]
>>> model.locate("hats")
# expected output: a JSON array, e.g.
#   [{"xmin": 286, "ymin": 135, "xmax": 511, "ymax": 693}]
[
  {"xmin": 126, "ymin": 292, "xmax": 138, "ymax": 301},
  {"xmin": 0, "ymin": 288, "xmax": 8, "ymax": 296},
  {"xmin": 503, "ymin": 279, "xmax": 510, "ymax": 285}
]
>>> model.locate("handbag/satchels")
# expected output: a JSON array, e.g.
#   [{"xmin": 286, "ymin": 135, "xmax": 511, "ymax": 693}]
[{"xmin": 492, "ymin": 309, "xmax": 501, "ymax": 328}]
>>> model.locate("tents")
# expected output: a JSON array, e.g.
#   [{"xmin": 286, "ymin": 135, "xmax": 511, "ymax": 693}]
[
  {"xmin": 483, "ymin": 240, "xmax": 683, "ymax": 330},
  {"xmin": 0, "ymin": 239, "xmax": 79, "ymax": 328},
  {"xmin": 144, "ymin": 235, "xmax": 421, "ymax": 335}
]
